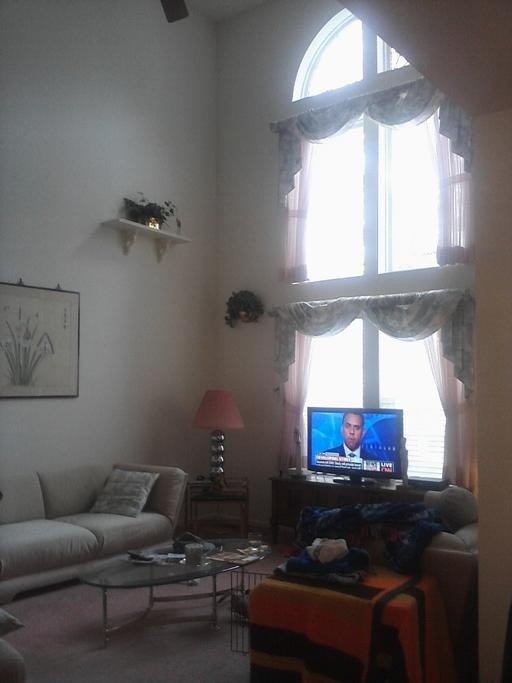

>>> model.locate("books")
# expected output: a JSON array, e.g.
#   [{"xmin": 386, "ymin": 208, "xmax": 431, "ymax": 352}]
[{"xmin": 204, "ymin": 550, "xmax": 259, "ymax": 565}]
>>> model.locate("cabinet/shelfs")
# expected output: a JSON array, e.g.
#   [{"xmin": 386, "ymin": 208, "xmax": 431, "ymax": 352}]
[{"xmin": 266, "ymin": 473, "xmax": 450, "ymax": 543}]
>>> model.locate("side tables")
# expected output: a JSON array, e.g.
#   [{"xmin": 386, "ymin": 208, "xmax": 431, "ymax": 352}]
[{"xmin": 185, "ymin": 476, "xmax": 250, "ymax": 538}]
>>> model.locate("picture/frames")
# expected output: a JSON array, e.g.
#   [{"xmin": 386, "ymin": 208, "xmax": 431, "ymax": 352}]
[{"xmin": 0, "ymin": 278, "xmax": 80, "ymax": 398}]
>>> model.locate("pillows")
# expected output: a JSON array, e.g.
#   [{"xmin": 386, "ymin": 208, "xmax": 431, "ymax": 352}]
[{"xmin": 88, "ymin": 467, "xmax": 160, "ymax": 517}]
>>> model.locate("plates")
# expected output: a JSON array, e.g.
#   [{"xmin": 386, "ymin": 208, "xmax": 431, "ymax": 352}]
[{"xmin": 179, "ymin": 557, "xmax": 209, "ymax": 567}]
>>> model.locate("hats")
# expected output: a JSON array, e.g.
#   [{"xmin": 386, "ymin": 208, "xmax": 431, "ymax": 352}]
[{"xmin": 424, "ymin": 486, "xmax": 478, "ymax": 524}]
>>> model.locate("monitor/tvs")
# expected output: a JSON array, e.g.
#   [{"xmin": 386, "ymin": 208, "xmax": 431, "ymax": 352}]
[{"xmin": 305, "ymin": 406, "xmax": 404, "ymax": 488}]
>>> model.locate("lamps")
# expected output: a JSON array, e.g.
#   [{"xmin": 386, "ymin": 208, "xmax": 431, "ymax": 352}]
[{"xmin": 190, "ymin": 390, "xmax": 245, "ymax": 495}]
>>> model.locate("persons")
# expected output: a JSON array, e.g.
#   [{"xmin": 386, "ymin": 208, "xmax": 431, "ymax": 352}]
[{"xmin": 323, "ymin": 409, "xmax": 382, "ymax": 460}]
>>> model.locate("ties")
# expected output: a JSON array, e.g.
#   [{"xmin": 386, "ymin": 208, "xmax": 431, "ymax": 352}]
[{"xmin": 349, "ymin": 453, "xmax": 355, "ymax": 457}]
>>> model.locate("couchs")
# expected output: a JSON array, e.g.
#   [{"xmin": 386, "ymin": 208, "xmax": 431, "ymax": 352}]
[
  {"xmin": 0, "ymin": 464, "xmax": 189, "ymax": 605},
  {"xmin": 244, "ymin": 486, "xmax": 478, "ymax": 683}
]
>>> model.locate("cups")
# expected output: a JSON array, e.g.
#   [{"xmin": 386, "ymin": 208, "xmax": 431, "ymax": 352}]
[
  {"xmin": 247, "ymin": 532, "xmax": 264, "ymax": 551},
  {"xmin": 184, "ymin": 543, "xmax": 203, "ymax": 565}
]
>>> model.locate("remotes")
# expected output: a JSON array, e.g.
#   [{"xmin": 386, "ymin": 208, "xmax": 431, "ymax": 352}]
[{"xmin": 126, "ymin": 547, "xmax": 154, "ymax": 561}]
